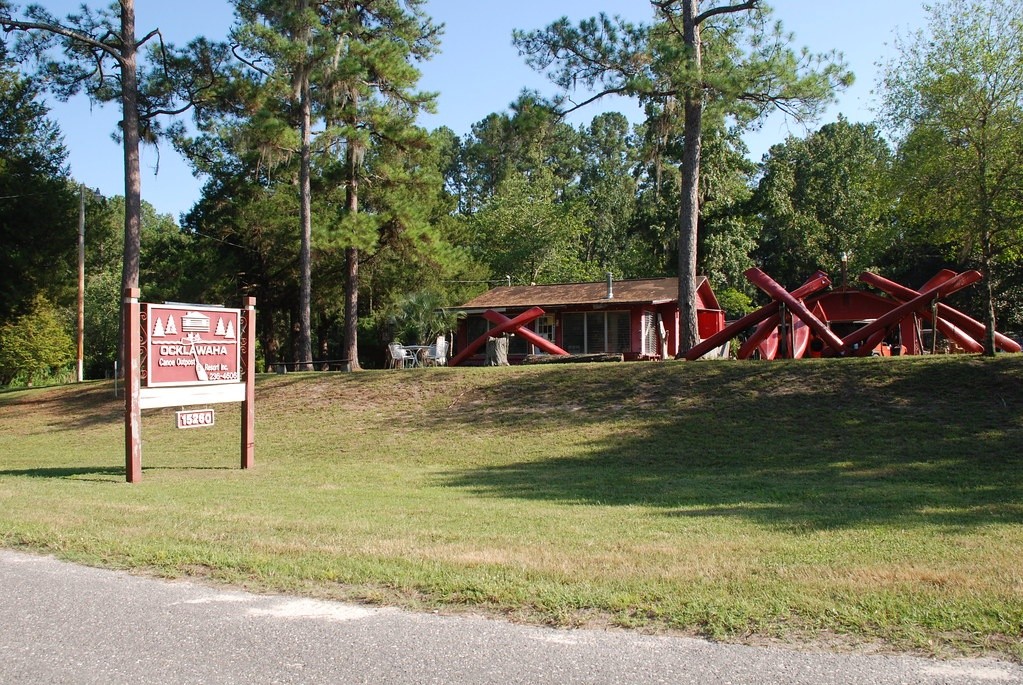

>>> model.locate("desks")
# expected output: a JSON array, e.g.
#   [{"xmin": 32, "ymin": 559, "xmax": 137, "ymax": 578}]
[{"xmin": 400, "ymin": 346, "xmax": 433, "ymax": 369}]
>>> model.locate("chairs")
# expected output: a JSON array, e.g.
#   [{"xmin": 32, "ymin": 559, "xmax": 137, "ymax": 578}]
[
  {"xmin": 428, "ymin": 344, "xmax": 436, "ymax": 359},
  {"xmin": 429, "ymin": 341, "xmax": 449, "ymax": 367},
  {"xmin": 391, "ymin": 345, "xmax": 414, "ymax": 368},
  {"xmin": 388, "ymin": 342, "xmax": 407, "ymax": 370}
]
